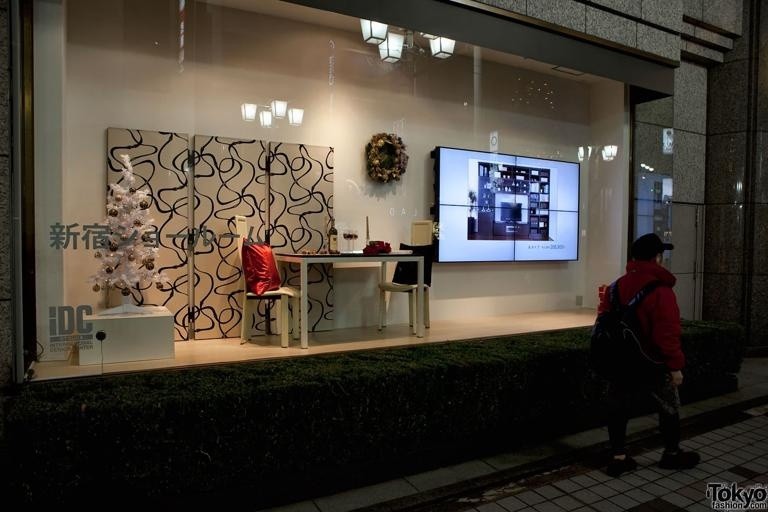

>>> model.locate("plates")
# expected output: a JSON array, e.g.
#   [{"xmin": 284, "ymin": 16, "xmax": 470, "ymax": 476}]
[{"xmin": 337, "ymin": 249, "xmax": 365, "ymax": 256}]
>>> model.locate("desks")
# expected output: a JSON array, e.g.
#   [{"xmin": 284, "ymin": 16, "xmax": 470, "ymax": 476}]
[{"xmin": 273, "ymin": 252, "xmax": 425, "ymax": 349}]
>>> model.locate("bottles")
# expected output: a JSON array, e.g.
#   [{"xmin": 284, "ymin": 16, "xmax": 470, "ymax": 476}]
[{"xmin": 328, "ymin": 217, "xmax": 340, "ymax": 254}]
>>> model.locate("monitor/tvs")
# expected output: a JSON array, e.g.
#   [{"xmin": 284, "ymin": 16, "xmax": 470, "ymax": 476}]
[{"xmin": 500, "ymin": 202, "xmax": 522, "ymax": 223}]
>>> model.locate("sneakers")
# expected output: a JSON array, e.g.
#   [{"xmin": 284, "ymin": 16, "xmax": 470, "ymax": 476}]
[
  {"xmin": 659, "ymin": 451, "xmax": 700, "ymax": 469},
  {"xmin": 606, "ymin": 455, "xmax": 637, "ymax": 477}
]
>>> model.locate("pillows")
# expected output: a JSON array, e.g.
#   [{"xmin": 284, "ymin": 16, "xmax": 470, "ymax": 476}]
[
  {"xmin": 242, "ymin": 237, "xmax": 282, "ymax": 295},
  {"xmin": 392, "ymin": 241, "xmax": 435, "ymax": 288}
]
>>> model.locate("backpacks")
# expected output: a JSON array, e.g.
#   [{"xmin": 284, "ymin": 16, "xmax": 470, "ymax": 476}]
[{"xmin": 590, "ymin": 281, "xmax": 657, "ymax": 379}]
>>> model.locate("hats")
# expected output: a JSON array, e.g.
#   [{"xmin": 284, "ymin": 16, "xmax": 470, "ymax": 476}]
[{"xmin": 632, "ymin": 233, "xmax": 673, "ymax": 259}]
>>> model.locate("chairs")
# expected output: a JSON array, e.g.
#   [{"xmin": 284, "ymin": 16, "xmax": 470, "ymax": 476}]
[
  {"xmin": 377, "ymin": 220, "xmax": 434, "ymax": 335},
  {"xmin": 235, "ymin": 215, "xmax": 300, "ymax": 348}
]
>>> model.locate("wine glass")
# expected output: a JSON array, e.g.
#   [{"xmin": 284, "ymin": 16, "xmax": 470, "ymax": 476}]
[{"xmin": 341, "ymin": 230, "xmax": 360, "ymax": 250}]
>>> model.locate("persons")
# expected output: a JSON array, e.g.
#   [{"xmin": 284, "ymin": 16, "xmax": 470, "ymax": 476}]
[{"xmin": 587, "ymin": 231, "xmax": 701, "ymax": 480}]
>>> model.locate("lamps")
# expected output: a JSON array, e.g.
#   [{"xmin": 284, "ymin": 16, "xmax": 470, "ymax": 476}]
[
  {"xmin": 242, "ymin": 96, "xmax": 306, "ymax": 131},
  {"xmin": 578, "ymin": 136, "xmax": 618, "ymax": 163},
  {"xmin": 359, "ymin": 19, "xmax": 457, "ymax": 64}
]
